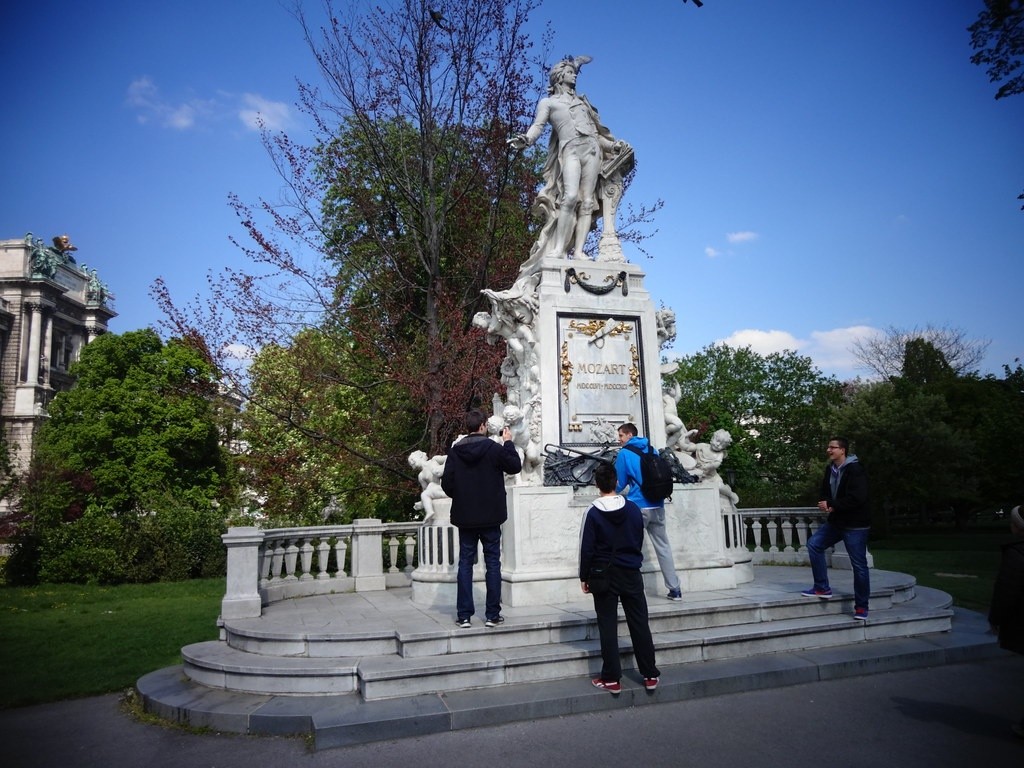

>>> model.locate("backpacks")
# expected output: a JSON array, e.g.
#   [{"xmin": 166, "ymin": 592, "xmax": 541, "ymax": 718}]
[{"xmin": 623, "ymin": 444, "xmax": 673, "ymax": 500}]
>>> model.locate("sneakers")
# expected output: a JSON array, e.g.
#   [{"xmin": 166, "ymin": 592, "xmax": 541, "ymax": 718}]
[
  {"xmin": 644, "ymin": 677, "xmax": 659, "ymax": 689},
  {"xmin": 801, "ymin": 587, "xmax": 832, "ymax": 598},
  {"xmin": 592, "ymin": 677, "xmax": 621, "ymax": 693},
  {"xmin": 485, "ymin": 616, "xmax": 504, "ymax": 626},
  {"xmin": 854, "ymin": 608, "xmax": 868, "ymax": 619},
  {"xmin": 456, "ymin": 618, "xmax": 471, "ymax": 628},
  {"xmin": 667, "ymin": 590, "xmax": 682, "ymax": 600}
]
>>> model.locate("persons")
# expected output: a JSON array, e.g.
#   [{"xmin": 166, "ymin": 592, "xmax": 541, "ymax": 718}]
[
  {"xmin": 471, "ymin": 290, "xmax": 535, "ymax": 364},
  {"xmin": 408, "ymin": 450, "xmax": 447, "ymax": 524},
  {"xmin": 989, "ymin": 505, "xmax": 1024, "ymax": 741},
  {"xmin": 504, "ymin": 300, "xmax": 536, "ymax": 326},
  {"xmin": 801, "ymin": 437, "xmax": 872, "ymax": 619},
  {"xmin": 441, "ymin": 410, "xmax": 521, "ymax": 627},
  {"xmin": 579, "ymin": 463, "xmax": 661, "ymax": 693},
  {"xmin": 663, "ymin": 377, "xmax": 739, "ymax": 504},
  {"xmin": 488, "ymin": 397, "xmax": 538, "ymax": 478},
  {"xmin": 615, "ymin": 423, "xmax": 682, "ymax": 601},
  {"xmin": 506, "ymin": 61, "xmax": 625, "ymax": 263}
]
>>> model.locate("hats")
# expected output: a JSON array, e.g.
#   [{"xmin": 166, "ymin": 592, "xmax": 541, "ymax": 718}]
[{"xmin": 1011, "ymin": 505, "xmax": 1024, "ymax": 528}]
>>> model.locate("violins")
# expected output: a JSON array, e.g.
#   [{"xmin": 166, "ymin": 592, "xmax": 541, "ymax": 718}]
[{"xmin": 571, "ymin": 446, "xmax": 610, "ymax": 483}]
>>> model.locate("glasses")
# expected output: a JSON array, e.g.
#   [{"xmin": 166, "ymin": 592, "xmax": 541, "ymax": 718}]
[{"xmin": 827, "ymin": 446, "xmax": 840, "ymax": 449}]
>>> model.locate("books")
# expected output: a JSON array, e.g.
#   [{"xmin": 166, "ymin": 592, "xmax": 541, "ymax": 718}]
[{"xmin": 602, "ymin": 144, "xmax": 635, "ymax": 179}]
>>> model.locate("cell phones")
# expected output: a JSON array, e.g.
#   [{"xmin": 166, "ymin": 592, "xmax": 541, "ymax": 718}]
[{"xmin": 499, "ymin": 431, "xmax": 503, "ymax": 436}]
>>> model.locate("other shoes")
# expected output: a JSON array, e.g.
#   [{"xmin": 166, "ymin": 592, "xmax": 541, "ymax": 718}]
[{"xmin": 1012, "ymin": 724, "xmax": 1024, "ymax": 736}]
